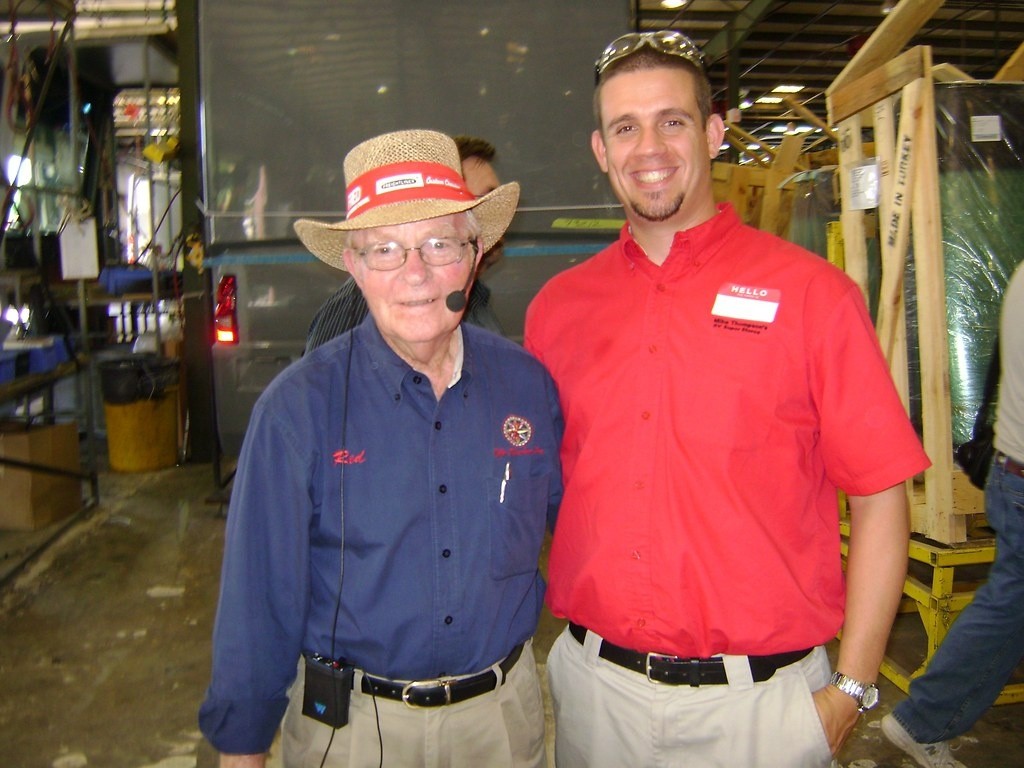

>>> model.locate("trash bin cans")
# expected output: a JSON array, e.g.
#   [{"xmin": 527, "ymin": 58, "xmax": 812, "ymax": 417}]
[{"xmin": 97, "ymin": 353, "xmax": 189, "ymax": 472}]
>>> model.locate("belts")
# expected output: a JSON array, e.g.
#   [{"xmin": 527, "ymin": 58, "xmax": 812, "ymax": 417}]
[
  {"xmin": 568, "ymin": 621, "xmax": 813, "ymax": 686},
  {"xmin": 996, "ymin": 452, "xmax": 1024, "ymax": 478},
  {"xmin": 351, "ymin": 642, "xmax": 524, "ymax": 710}
]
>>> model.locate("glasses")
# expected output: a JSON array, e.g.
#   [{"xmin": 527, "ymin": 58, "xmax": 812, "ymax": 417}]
[
  {"xmin": 353, "ymin": 237, "xmax": 472, "ymax": 271},
  {"xmin": 595, "ymin": 30, "xmax": 702, "ymax": 85}
]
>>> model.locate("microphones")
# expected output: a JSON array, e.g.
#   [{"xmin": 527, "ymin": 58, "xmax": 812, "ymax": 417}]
[{"xmin": 447, "ymin": 241, "xmax": 479, "ymax": 314}]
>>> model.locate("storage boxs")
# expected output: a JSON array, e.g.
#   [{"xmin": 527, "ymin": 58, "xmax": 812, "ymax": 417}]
[{"xmin": 0, "ymin": 422, "xmax": 82, "ymax": 532}]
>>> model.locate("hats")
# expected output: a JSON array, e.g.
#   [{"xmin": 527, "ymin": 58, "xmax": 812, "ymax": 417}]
[{"xmin": 293, "ymin": 130, "xmax": 520, "ymax": 273}]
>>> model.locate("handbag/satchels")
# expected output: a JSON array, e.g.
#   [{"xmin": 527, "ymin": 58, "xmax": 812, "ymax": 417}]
[{"xmin": 954, "ymin": 424, "xmax": 995, "ymax": 490}]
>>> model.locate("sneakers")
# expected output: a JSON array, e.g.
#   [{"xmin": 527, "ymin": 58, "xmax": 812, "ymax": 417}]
[{"xmin": 882, "ymin": 713, "xmax": 967, "ymax": 768}]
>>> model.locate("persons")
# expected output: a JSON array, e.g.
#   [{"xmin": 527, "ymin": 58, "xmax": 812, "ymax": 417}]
[
  {"xmin": 523, "ymin": 31, "xmax": 932, "ymax": 768},
  {"xmin": 197, "ymin": 129, "xmax": 566, "ymax": 768},
  {"xmin": 882, "ymin": 265, "xmax": 1024, "ymax": 768}
]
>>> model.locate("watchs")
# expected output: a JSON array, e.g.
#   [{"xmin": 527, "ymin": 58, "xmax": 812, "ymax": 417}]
[{"xmin": 830, "ymin": 671, "xmax": 879, "ymax": 712}]
